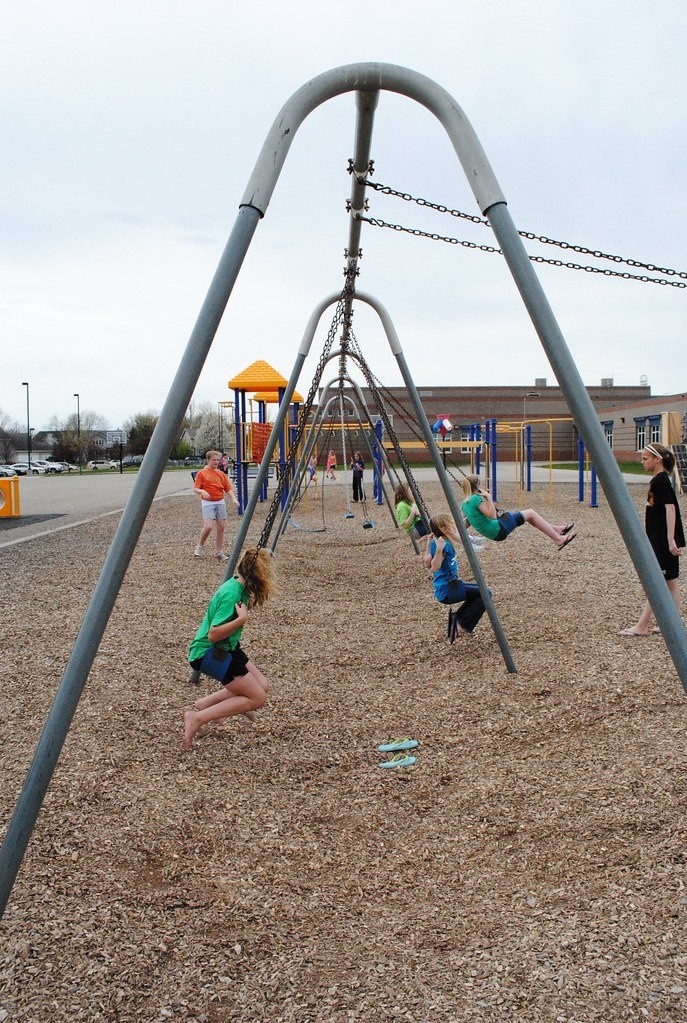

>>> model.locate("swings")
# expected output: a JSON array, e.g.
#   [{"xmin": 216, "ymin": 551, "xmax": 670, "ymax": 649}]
[
  {"xmin": 286, "ymin": 380, "xmax": 338, "ymax": 533},
  {"xmin": 341, "ymin": 369, "xmax": 373, "ymax": 529},
  {"xmin": 346, "ymin": 345, "xmax": 507, "ymax": 540},
  {"xmin": 344, "ymin": 370, "xmax": 430, "ymax": 538},
  {"xmin": 339, "ymin": 381, "xmax": 354, "ymax": 518},
  {"xmin": 354, "ymin": 176, "xmax": 687, "ymax": 288},
  {"xmin": 197, "ymin": 271, "xmax": 353, "ymax": 680},
  {"xmin": 347, "ymin": 328, "xmax": 459, "ymax": 605}
]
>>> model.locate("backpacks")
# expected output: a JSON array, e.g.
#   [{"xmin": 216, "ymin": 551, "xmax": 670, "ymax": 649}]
[{"xmin": 219, "ymin": 462, "xmax": 224, "ymax": 471}]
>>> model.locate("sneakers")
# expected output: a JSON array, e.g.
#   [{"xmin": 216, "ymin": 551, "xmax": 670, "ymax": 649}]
[
  {"xmin": 194, "ymin": 544, "xmax": 203, "ymax": 557},
  {"xmin": 214, "ymin": 551, "xmax": 229, "ymax": 563}
]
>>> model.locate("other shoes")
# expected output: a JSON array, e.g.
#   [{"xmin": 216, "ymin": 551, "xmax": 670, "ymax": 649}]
[
  {"xmin": 469, "ymin": 535, "xmax": 484, "ymax": 543},
  {"xmin": 472, "ymin": 544, "xmax": 484, "ymax": 553},
  {"xmin": 330, "ymin": 477, "xmax": 333, "ymax": 479},
  {"xmin": 350, "ymin": 500, "xmax": 357, "ymax": 503},
  {"xmin": 326, "ymin": 474, "xmax": 328, "ymax": 478},
  {"xmin": 360, "ymin": 500, "xmax": 365, "ymax": 503}
]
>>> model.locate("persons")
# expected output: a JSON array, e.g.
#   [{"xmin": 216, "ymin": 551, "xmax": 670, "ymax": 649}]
[
  {"xmin": 395, "ymin": 484, "xmax": 487, "ymax": 557},
  {"xmin": 307, "ymin": 454, "xmax": 316, "ymax": 480},
  {"xmin": 326, "ymin": 451, "xmax": 337, "ymax": 481},
  {"xmin": 617, "ymin": 443, "xmax": 687, "ymax": 637},
  {"xmin": 425, "ymin": 514, "xmax": 492, "ymax": 645},
  {"xmin": 350, "ymin": 451, "xmax": 366, "ymax": 504},
  {"xmin": 194, "ymin": 450, "xmax": 240, "ymax": 559},
  {"xmin": 184, "ymin": 549, "xmax": 271, "ymax": 751},
  {"xmin": 461, "ymin": 475, "xmax": 577, "ymax": 551}
]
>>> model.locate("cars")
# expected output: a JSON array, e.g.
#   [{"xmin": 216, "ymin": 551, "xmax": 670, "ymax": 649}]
[
  {"xmin": 87, "ymin": 460, "xmax": 117, "ymax": 470},
  {"xmin": 110, "ymin": 454, "xmax": 144, "ymax": 468},
  {"xmin": 0, "ymin": 459, "xmax": 79, "ymax": 477}
]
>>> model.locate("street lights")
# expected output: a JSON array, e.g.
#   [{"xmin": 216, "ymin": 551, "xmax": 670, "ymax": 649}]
[
  {"xmin": 74, "ymin": 394, "xmax": 82, "ymax": 475},
  {"xmin": 21, "ymin": 382, "xmax": 33, "ymax": 475}
]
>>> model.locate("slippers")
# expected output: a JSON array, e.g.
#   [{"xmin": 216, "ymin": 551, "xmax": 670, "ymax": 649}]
[
  {"xmin": 652, "ymin": 628, "xmax": 661, "ymax": 634},
  {"xmin": 559, "ymin": 534, "xmax": 577, "ymax": 550},
  {"xmin": 450, "ymin": 613, "xmax": 463, "ymax": 644},
  {"xmin": 379, "ymin": 738, "xmax": 418, "ymax": 751},
  {"xmin": 560, "ymin": 524, "xmax": 574, "ymax": 535},
  {"xmin": 379, "ymin": 753, "xmax": 416, "ymax": 768},
  {"xmin": 617, "ymin": 628, "xmax": 651, "ymax": 637},
  {"xmin": 447, "ymin": 608, "xmax": 453, "ymax": 638}
]
